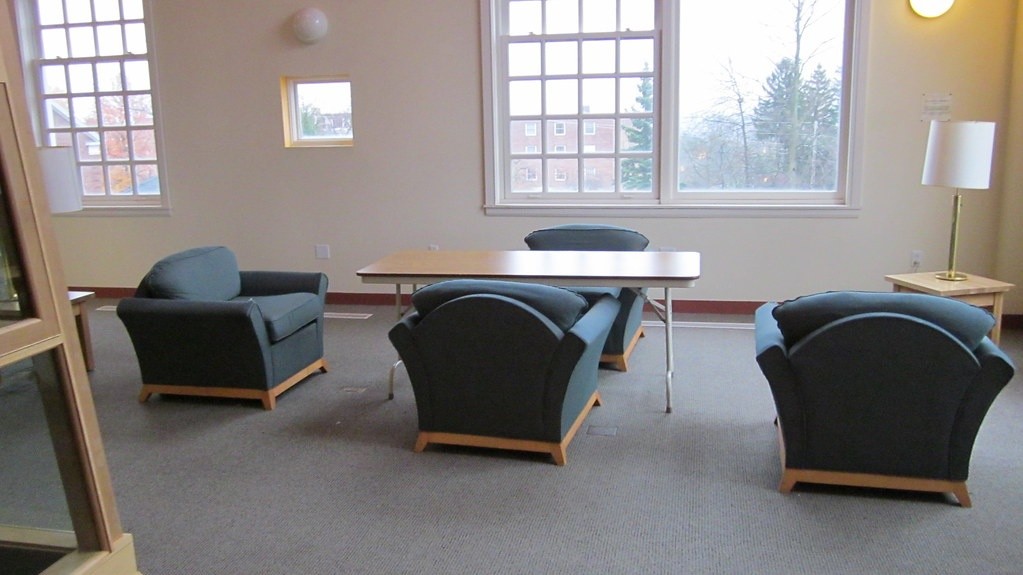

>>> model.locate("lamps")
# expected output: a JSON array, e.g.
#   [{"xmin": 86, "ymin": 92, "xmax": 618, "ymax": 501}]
[
  {"xmin": 919, "ymin": 119, "xmax": 998, "ymax": 281},
  {"xmin": 35, "ymin": 144, "xmax": 84, "ymax": 217}
]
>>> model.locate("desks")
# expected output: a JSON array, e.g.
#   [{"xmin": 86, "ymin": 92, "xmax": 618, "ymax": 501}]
[
  {"xmin": 356, "ymin": 248, "xmax": 701, "ymax": 413},
  {"xmin": 1, "ymin": 289, "xmax": 97, "ymax": 375},
  {"xmin": 883, "ymin": 271, "xmax": 1017, "ymax": 349}
]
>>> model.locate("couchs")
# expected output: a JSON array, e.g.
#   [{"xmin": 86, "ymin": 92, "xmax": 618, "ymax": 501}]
[
  {"xmin": 386, "ymin": 277, "xmax": 623, "ymax": 467},
  {"xmin": 751, "ymin": 292, "xmax": 1017, "ymax": 508},
  {"xmin": 522, "ymin": 222, "xmax": 651, "ymax": 372},
  {"xmin": 116, "ymin": 243, "xmax": 331, "ymax": 412}
]
